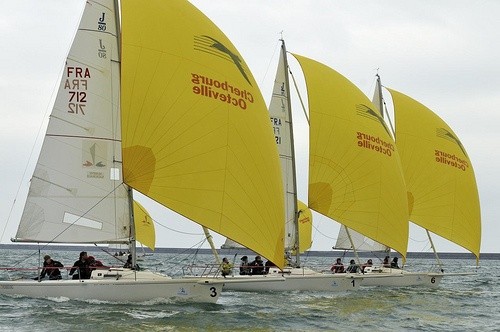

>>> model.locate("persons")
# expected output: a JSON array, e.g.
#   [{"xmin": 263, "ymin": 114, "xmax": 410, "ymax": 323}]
[
  {"xmin": 124, "ymin": 255, "xmax": 140, "ymax": 271},
  {"xmin": 219, "ymin": 256, "xmax": 276, "ymax": 276},
  {"xmin": 34, "ymin": 255, "xmax": 64, "ymax": 280},
  {"xmin": 331, "ymin": 255, "xmax": 399, "ymax": 273},
  {"xmin": 68, "ymin": 252, "xmax": 103, "ymax": 279}
]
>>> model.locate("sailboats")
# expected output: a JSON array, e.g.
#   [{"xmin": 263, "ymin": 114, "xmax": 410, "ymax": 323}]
[
  {"xmin": 0, "ymin": 0, "xmax": 286, "ymax": 305},
  {"xmin": 194, "ymin": 38, "xmax": 410, "ymax": 294},
  {"xmin": 323, "ymin": 72, "xmax": 482, "ymax": 288}
]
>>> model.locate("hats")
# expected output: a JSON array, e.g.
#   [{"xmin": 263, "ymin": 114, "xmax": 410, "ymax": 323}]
[
  {"xmin": 87, "ymin": 256, "xmax": 95, "ymax": 261},
  {"xmin": 44, "ymin": 255, "xmax": 50, "ymax": 261}
]
